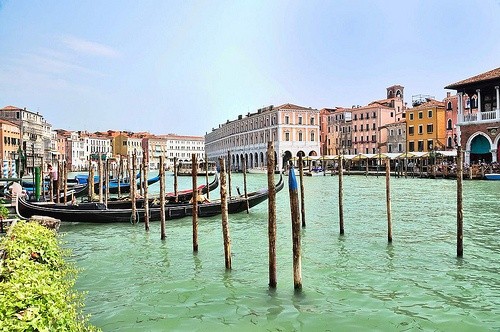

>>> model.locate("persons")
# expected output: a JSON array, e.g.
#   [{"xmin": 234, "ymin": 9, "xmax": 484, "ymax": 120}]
[
  {"xmin": 287, "ymin": 158, "xmax": 290, "ymax": 171},
  {"xmin": 393, "ymin": 159, "xmax": 499, "ymax": 173},
  {"xmin": 335, "ymin": 162, "xmax": 338, "ymax": 172},
  {"xmin": 47, "ymin": 164, "xmax": 57, "ymax": 197},
  {"xmin": 318, "ymin": 164, "xmax": 322, "ymax": 169},
  {"xmin": 4, "ymin": 186, "xmax": 12, "ymax": 195},
  {"xmin": 190, "ymin": 189, "xmax": 209, "ymax": 204}
]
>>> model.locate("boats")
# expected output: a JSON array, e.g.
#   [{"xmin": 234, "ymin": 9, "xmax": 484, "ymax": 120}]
[
  {"xmin": 279, "ymin": 164, "xmax": 337, "ymax": 179},
  {"xmin": 0, "ymin": 157, "xmax": 219, "ymax": 218},
  {"xmin": 17, "ymin": 172, "xmax": 284, "ymax": 222},
  {"xmin": 246, "ymin": 165, "xmax": 269, "ymax": 174}
]
